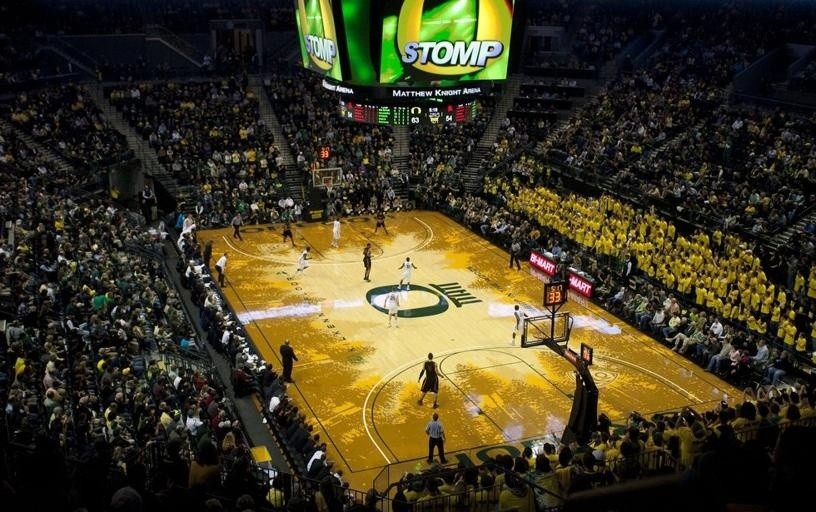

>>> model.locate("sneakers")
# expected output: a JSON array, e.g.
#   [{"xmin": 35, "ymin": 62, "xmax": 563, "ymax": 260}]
[
  {"xmin": 284, "ymin": 379, "xmax": 294, "ymax": 382},
  {"xmin": 397, "ymin": 287, "xmax": 410, "ymax": 291},
  {"xmin": 509, "ymin": 341, "xmax": 515, "ymax": 345},
  {"xmin": 417, "ymin": 399, "xmax": 439, "ymax": 409}
]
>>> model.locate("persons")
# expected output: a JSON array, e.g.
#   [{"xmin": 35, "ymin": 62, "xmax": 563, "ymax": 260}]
[{"xmin": 0, "ymin": 0, "xmax": 816, "ymax": 512}]
